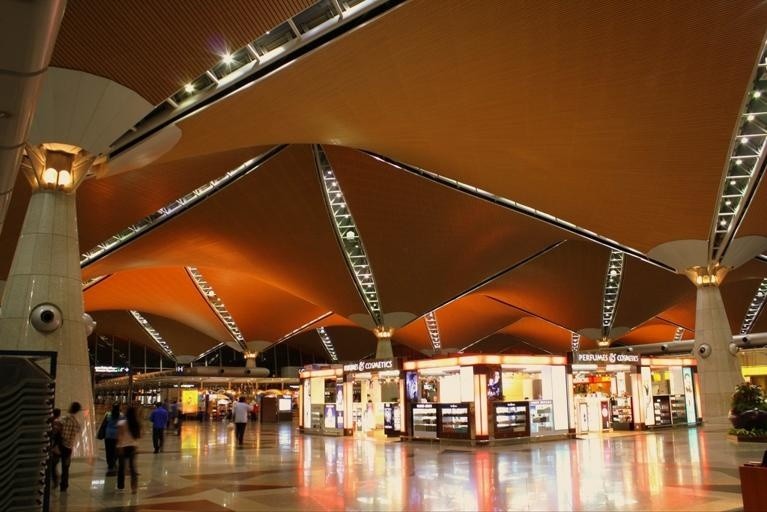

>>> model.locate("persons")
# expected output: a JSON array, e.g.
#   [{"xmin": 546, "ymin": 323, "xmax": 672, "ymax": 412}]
[
  {"xmin": 153, "ymin": 398, "xmax": 185, "ymax": 436},
  {"xmin": 487, "ymin": 368, "xmax": 499, "ymax": 398},
  {"xmin": 49, "ymin": 401, "xmax": 82, "ymax": 492},
  {"xmin": 149, "ymin": 401, "xmax": 168, "ymax": 453},
  {"xmin": 336, "ymin": 386, "xmax": 342, "ymax": 410},
  {"xmin": 684, "ymin": 372, "xmax": 693, "ymax": 405},
  {"xmin": 49, "ymin": 408, "xmax": 60, "ymax": 424},
  {"xmin": 231, "ymin": 395, "xmax": 253, "ymax": 448},
  {"xmin": 96, "ymin": 403, "xmax": 124, "ymax": 476},
  {"xmin": 114, "ymin": 405, "xmax": 142, "ymax": 494}
]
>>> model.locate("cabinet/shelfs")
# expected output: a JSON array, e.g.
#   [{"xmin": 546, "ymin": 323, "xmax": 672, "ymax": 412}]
[
  {"xmin": 307, "ymin": 394, "xmax": 688, "ymax": 445},
  {"xmin": 204, "ymin": 393, "xmax": 234, "ymax": 421}
]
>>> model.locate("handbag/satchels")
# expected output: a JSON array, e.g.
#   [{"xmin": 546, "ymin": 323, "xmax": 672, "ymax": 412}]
[
  {"xmin": 94, "ymin": 412, "xmax": 110, "ymax": 439},
  {"xmin": 231, "ymin": 412, "xmax": 236, "ymax": 422}
]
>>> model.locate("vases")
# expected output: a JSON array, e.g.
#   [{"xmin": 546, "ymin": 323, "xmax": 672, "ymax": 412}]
[
  {"xmin": 727, "ymin": 406, "xmax": 766, "ymax": 431},
  {"xmin": 739, "ymin": 463, "xmax": 766, "ymax": 510}
]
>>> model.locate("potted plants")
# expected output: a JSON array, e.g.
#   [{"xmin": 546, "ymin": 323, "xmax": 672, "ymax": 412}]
[{"xmin": 726, "ymin": 427, "xmax": 767, "ymax": 441}]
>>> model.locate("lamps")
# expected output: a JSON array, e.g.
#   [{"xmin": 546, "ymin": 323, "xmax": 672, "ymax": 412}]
[
  {"xmin": 39, "ymin": 166, "xmax": 79, "ymax": 191},
  {"xmin": 695, "ymin": 274, "xmax": 718, "ymax": 288}
]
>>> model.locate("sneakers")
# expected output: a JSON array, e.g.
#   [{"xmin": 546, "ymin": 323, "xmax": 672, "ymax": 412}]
[
  {"xmin": 51, "ymin": 480, "xmax": 58, "ymax": 489},
  {"xmin": 114, "ymin": 484, "xmax": 125, "ymax": 494},
  {"xmin": 238, "ymin": 442, "xmax": 244, "ymax": 447},
  {"xmin": 130, "ymin": 489, "xmax": 138, "ymax": 495},
  {"xmin": 153, "ymin": 446, "xmax": 160, "ymax": 454},
  {"xmin": 60, "ymin": 483, "xmax": 69, "ymax": 493},
  {"xmin": 159, "ymin": 445, "xmax": 164, "ymax": 452}
]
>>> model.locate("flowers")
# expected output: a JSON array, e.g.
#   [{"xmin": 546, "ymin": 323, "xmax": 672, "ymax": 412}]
[{"xmin": 729, "ymin": 382, "xmax": 764, "ymax": 411}]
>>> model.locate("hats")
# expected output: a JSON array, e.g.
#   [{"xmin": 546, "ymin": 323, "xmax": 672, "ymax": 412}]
[{"xmin": 238, "ymin": 396, "xmax": 245, "ymax": 402}]
